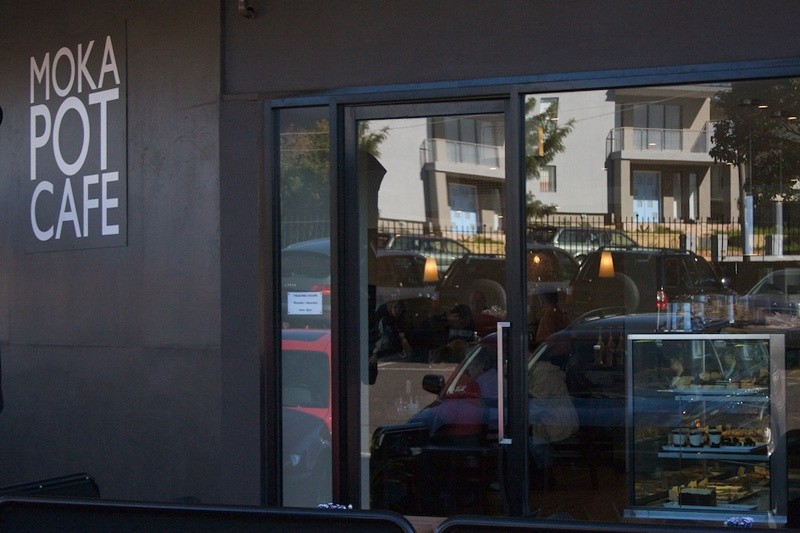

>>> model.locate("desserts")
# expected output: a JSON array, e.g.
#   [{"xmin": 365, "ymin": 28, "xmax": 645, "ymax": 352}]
[
  {"xmin": 721, "ymin": 429, "xmax": 765, "ymax": 446},
  {"xmin": 672, "ymin": 371, "xmax": 752, "ymax": 390}
]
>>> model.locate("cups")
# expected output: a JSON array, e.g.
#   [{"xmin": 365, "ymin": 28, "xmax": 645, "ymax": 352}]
[
  {"xmin": 671, "ymin": 426, "xmax": 721, "ymax": 448},
  {"xmin": 666, "ymin": 293, "xmax": 735, "ymax": 330}
]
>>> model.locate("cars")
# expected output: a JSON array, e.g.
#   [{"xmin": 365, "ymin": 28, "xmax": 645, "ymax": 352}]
[
  {"xmin": 281, "ymin": 236, "xmax": 331, "ymax": 440},
  {"xmin": 368, "ymin": 231, "xmax": 800, "ymax": 517}
]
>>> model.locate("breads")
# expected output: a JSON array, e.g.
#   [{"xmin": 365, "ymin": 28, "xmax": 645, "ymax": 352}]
[{"xmin": 669, "ymin": 475, "xmax": 768, "ymax": 501}]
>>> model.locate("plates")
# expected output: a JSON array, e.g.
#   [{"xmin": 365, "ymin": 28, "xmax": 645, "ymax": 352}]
[{"xmin": 664, "ymin": 501, "xmax": 757, "ymax": 511}]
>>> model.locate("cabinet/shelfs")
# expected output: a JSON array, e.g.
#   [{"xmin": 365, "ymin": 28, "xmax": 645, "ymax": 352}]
[{"xmin": 624, "ymin": 333, "xmax": 787, "ymax": 523}]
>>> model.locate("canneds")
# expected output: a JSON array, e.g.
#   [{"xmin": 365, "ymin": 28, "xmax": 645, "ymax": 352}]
[
  {"xmin": 672, "ymin": 428, "xmax": 705, "ymax": 448},
  {"xmin": 709, "ymin": 428, "xmax": 721, "ymax": 448}
]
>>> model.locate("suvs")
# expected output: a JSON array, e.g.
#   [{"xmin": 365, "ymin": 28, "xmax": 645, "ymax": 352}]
[{"xmin": 526, "ymin": 225, "xmax": 663, "ymax": 267}]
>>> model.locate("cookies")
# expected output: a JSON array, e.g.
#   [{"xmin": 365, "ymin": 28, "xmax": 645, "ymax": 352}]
[{"xmin": 690, "ymin": 301, "xmax": 756, "ymax": 322}]
